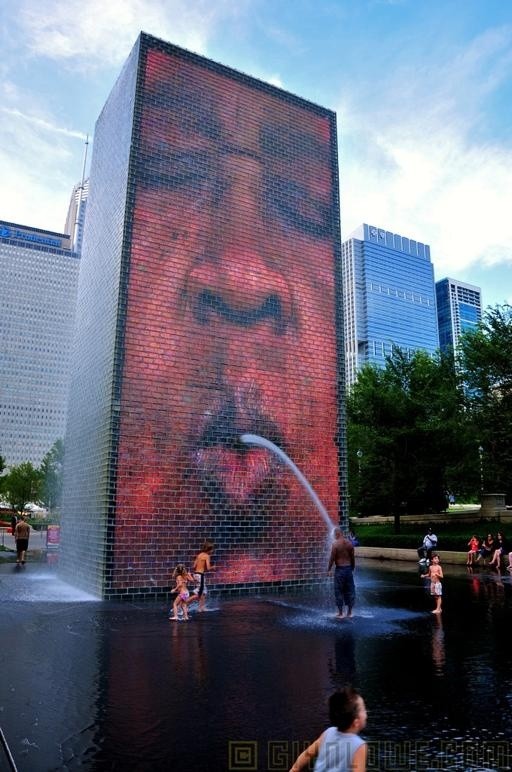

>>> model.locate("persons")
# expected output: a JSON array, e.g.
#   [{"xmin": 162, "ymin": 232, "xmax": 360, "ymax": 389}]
[
  {"xmin": 466, "ymin": 532, "xmax": 512, "ymax": 569},
  {"xmin": 289, "ymin": 688, "xmax": 368, "ymax": 772},
  {"xmin": 110, "ymin": 47, "xmax": 338, "ymax": 586},
  {"xmin": 327, "ymin": 527, "xmax": 356, "ymax": 619},
  {"xmin": 466, "ymin": 564, "xmax": 512, "ymax": 603},
  {"xmin": 14, "ymin": 516, "xmax": 31, "ymax": 564},
  {"xmin": 431, "ymin": 614, "xmax": 447, "ymax": 677},
  {"xmin": 168, "ymin": 563, "xmax": 194, "ymax": 622},
  {"xmin": 420, "ymin": 553, "xmax": 444, "ymax": 613},
  {"xmin": 170, "ymin": 619, "xmax": 207, "ymax": 688},
  {"xmin": 417, "ymin": 527, "xmax": 438, "ymax": 564},
  {"xmin": 11, "ymin": 513, "xmax": 18, "ymax": 536},
  {"xmin": 185, "ymin": 541, "xmax": 217, "ymax": 611}
]
